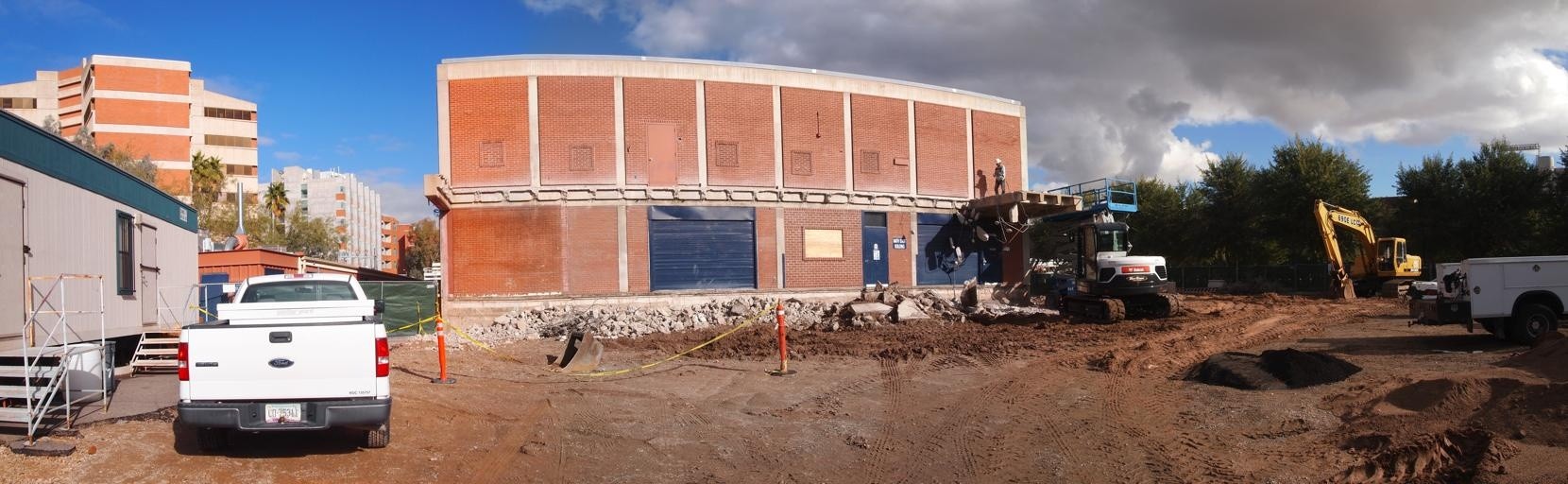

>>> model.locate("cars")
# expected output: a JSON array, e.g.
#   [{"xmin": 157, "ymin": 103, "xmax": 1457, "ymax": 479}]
[{"xmin": 1413, "ymin": 278, "xmax": 1437, "ymax": 301}]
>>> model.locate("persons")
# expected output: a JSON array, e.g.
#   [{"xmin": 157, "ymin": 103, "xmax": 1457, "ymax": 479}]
[{"xmin": 993, "ymin": 158, "xmax": 1005, "ymax": 195}]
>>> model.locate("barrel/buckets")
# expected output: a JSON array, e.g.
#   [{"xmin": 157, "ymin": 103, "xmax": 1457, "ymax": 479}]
[{"xmin": 60, "ymin": 341, "xmax": 104, "ymax": 404}]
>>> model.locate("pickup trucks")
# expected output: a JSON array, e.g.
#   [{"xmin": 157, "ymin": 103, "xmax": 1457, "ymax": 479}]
[{"xmin": 176, "ymin": 270, "xmax": 393, "ymax": 454}]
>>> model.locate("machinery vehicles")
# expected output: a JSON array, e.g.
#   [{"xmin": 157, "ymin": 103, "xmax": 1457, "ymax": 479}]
[
  {"xmin": 1030, "ymin": 212, "xmax": 1180, "ymax": 324},
  {"xmin": 1313, "ymin": 199, "xmax": 1423, "ymax": 299}
]
